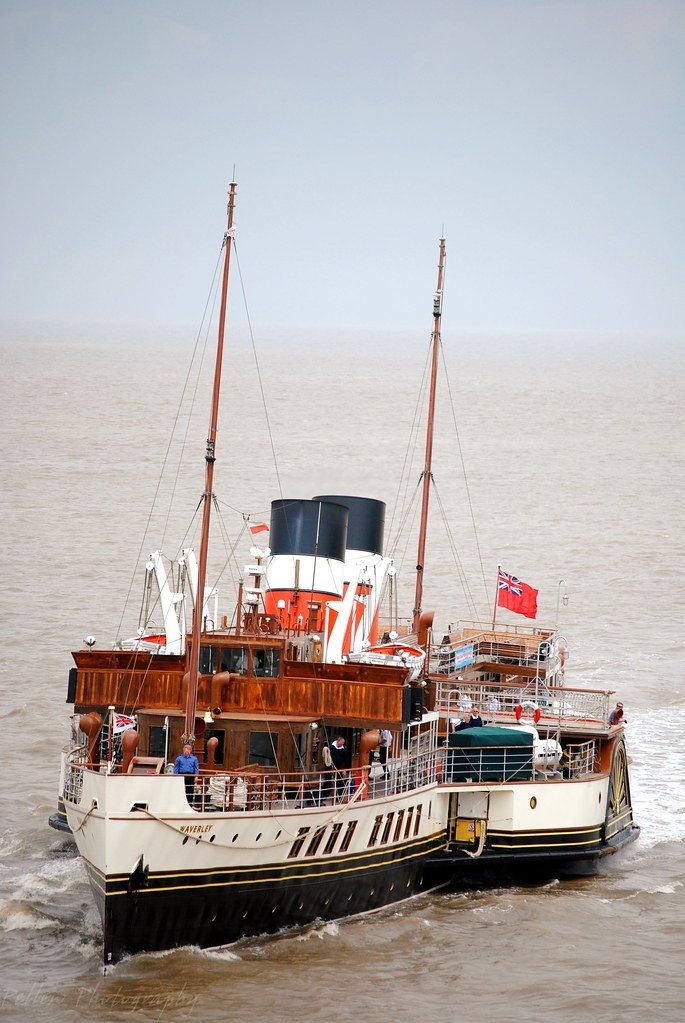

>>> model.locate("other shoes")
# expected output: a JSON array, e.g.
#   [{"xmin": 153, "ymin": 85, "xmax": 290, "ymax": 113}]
[
  {"xmin": 380, "ymin": 773, "xmax": 390, "ymax": 780},
  {"xmin": 368, "ymin": 776, "xmax": 374, "ymax": 780}
]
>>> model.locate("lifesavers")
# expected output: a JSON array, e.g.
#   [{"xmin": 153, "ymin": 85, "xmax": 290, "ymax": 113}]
[{"xmin": 516, "ymin": 700, "xmax": 541, "ymax": 726}]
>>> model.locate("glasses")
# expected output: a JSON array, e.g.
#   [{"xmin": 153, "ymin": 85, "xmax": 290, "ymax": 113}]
[{"xmin": 617, "ymin": 706, "xmax": 623, "ymax": 708}]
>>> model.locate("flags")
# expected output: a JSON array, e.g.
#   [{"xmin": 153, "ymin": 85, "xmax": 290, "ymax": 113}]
[
  {"xmin": 113, "ymin": 712, "xmax": 138, "ymax": 735},
  {"xmin": 496, "ymin": 569, "xmax": 540, "ymax": 619}
]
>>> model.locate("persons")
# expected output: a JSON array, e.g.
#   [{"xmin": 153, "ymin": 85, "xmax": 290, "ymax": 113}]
[
  {"xmin": 450, "ymin": 685, "xmax": 502, "ymax": 732},
  {"xmin": 173, "ymin": 744, "xmax": 199, "ymax": 807},
  {"xmin": 321, "ymin": 737, "xmax": 348, "ymax": 789},
  {"xmin": 609, "ymin": 702, "xmax": 627, "ymax": 725},
  {"xmin": 377, "ymin": 727, "xmax": 393, "ymax": 782}
]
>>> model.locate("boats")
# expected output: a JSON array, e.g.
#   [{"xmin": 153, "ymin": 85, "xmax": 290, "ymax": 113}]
[{"xmin": 49, "ymin": 164, "xmax": 641, "ymax": 974}]
